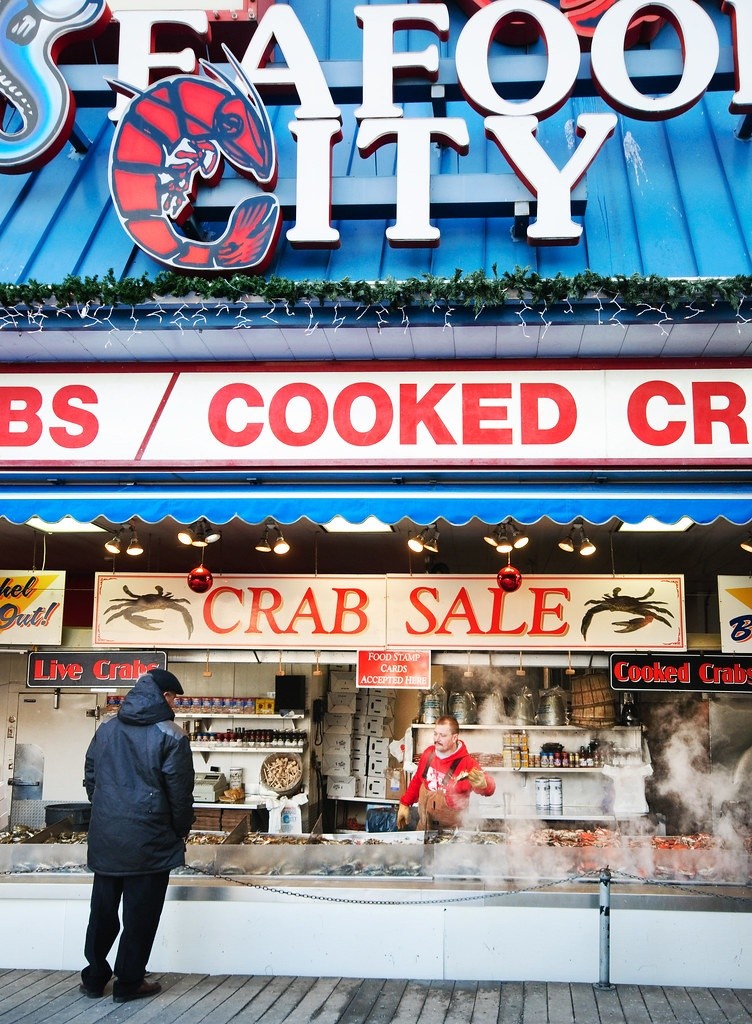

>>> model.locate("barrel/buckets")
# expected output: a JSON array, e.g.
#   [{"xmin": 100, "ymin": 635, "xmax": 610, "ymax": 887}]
[
  {"xmin": 44, "ymin": 804, "xmax": 92, "ymax": 834},
  {"xmin": 570, "ymin": 673, "xmax": 619, "ymax": 729}
]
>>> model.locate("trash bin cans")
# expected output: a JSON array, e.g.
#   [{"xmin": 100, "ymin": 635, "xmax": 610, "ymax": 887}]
[{"xmin": 45, "ymin": 803, "xmax": 92, "ymax": 832}]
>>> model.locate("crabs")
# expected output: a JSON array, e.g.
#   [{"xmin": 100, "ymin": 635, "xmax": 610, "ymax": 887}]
[
  {"xmin": 103, "ymin": 585, "xmax": 194, "ymax": 640},
  {"xmin": 625, "ymin": 832, "xmax": 726, "ymax": 881},
  {"xmin": 526, "ymin": 823, "xmax": 620, "ymax": 872},
  {"xmin": 580, "ymin": 587, "xmax": 675, "ymax": 642}
]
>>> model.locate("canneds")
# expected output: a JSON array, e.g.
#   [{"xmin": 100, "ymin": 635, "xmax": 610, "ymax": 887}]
[
  {"xmin": 535, "ymin": 777, "xmax": 563, "ymax": 808},
  {"xmin": 107, "ymin": 695, "xmax": 255, "ymax": 713},
  {"xmin": 502, "ymin": 733, "xmax": 569, "ymax": 768}
]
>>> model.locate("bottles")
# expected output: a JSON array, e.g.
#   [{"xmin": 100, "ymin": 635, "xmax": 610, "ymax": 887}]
[
  {"xmin": 527, "ymin": 745, "xmax": 641, "ymax": 768},
  {"xmin": 622, "ymin": 692, "xmax": 639, "ymax": 726},
  {"xmin": 179, "ymin": 719, "xmax": 309, "ymax": 749}
]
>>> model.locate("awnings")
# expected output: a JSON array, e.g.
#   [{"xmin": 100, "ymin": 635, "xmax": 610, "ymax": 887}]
[{"xmin": 0, "ymin": 461, "xmax": 751, "ymax": 526}]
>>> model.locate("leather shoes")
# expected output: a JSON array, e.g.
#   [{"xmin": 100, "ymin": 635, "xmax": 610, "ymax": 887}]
[
  {"xmin": 113, "ymin": 978, "xmax": 162, "ymax": 1002},
  {"xmin": 80, "ymin": 980, "xmax": 101, "ymax": 999}
]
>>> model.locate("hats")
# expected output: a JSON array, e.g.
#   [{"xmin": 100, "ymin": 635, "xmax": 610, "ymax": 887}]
[{"xmin": 147, "ymin": 667, "xmax": 184, "ymax": 695}]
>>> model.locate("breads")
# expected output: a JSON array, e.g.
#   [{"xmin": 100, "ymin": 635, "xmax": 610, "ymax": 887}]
[{"xmin": 219, "ymin": 787, "xmax": 245, "ymax": 802}]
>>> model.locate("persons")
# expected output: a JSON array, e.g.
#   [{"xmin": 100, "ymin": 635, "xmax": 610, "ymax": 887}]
[
  {"xmin": 396, "ymin": 716, "xmax": 496, "ymax": 832},
  {"xmin": 79, "ymin": 668, "xmax": 196, "ymax": 1003}
]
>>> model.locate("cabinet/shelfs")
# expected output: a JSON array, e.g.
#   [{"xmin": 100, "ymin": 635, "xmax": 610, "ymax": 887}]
[
  {"xmin": 105, "ymin": 713, "xmax": 309, "ymax": 809},
  {"xmin": 407, "ymin": 723, "xmax": 647, "ymax": 821}
]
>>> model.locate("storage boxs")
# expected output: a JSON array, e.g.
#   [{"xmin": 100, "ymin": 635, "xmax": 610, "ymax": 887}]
[{"xmin": 321, "ymin": 663, "xmax": 406, "ymax": 800}]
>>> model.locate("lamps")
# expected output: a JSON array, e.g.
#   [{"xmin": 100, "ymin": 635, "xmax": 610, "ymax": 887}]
[
  {"xmin": 558, "ymin": 517, "xmax": 597, "ymax": 556},
  {"xmin": 255, "ymin": 526, "xmax": 290, "ymax": 554},
  {"xmin": 740, "ymin": 536, "xmax": 752, "ymax": 553},
  {"xmin": 104, "ymin": 524, "xmax": 144, "ymax": 556},
  {"xmin": 483, "ymin": 518, "xmax": 529, "ymax": 554},
  {"xmin": 408, "ymin": 523, "xmax": 440, "ymax": 553},
  {"xmin": 177, "ymin": 519, "xmax": 222, "ymax": 546}
]
